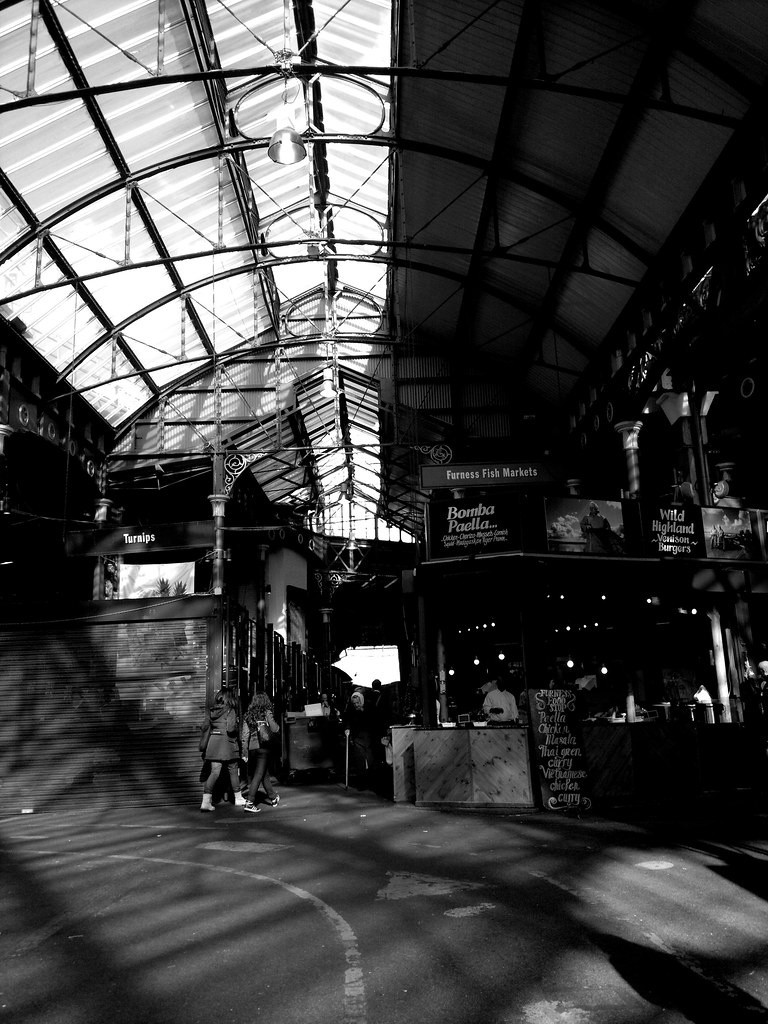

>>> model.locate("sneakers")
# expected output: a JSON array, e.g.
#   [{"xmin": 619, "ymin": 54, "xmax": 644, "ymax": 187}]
[
  {"xmin": 271, "ymin": 795, "xmax": 281, "ymax": 807},
  {"xmin": 243, "ymin": 803, "xmax": 262, "ymax": 812}
]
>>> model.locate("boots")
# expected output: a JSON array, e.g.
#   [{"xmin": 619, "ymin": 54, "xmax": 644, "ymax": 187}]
[
  {"xmin": 234, "ymin": 791, "xmax": 247, "ymax": 806},
  {"xmin": 200, "ymin": 793, "xmax": 215, "ymax": 811}
]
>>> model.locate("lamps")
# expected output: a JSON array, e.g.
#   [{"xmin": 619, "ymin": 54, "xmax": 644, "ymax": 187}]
[
  {"xmin": 320, "ymin": 340, "xmax": 339, "ymax": 398},
  {"xmin": 267, "ymin": 50, "xmax": 307, "ymax": 166},
  {"xmin": 447, "ymin": 589, "xmax": 705, "ymax": 682},
  {"xmin": 345, "ymin": 501, "xmax": 359, "ymax": 550}
]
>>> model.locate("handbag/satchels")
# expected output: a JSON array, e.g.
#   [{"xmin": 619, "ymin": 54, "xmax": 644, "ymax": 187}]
[
  {"xmin": 199, "ymin": 725, "xmax": 212, "ymax": 752},
  {"xmin": 257, "ymin": 724, "xmax": 277, "ymax": 748}
]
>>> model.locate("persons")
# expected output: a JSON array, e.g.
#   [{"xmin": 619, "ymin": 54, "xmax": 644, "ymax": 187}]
[
  {"xmin": 321, "ymin": 679, "xmax": 392, "ymax": 785},
  {"xmin": 241, "ymin": 690, "xmax": 280, "ymax": 813},
  {"xmin": 198, "ymin": 686, "xmax": 251, "ymax": 813},
  {"xmin": 579, "ymin": 501, "xmax": 611, "ymax": 556},
  {"xmin": 482, "ymin": 676, "xmax": 520, "ymax": 723},
  {"xmin": 710, "ymin": 524, "xmax": 726, "ymax": 550}
]
